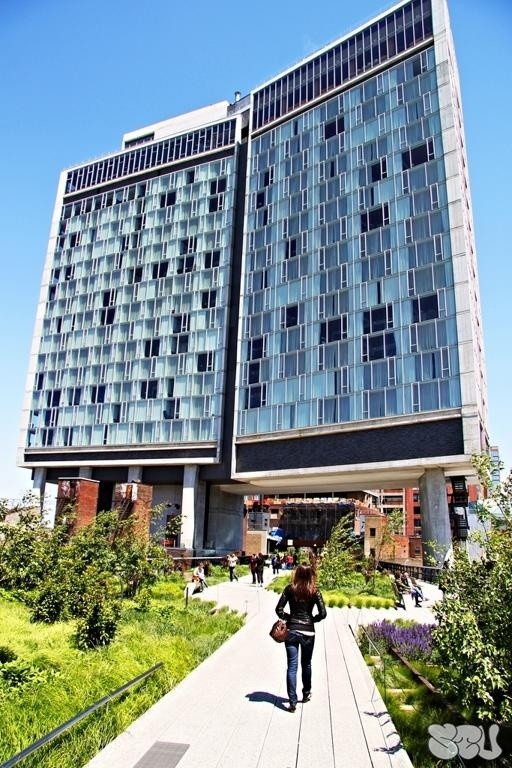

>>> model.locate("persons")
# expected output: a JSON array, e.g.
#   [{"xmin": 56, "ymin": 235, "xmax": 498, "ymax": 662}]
[
  {"xmin": 193, "ymin": 552, "xmax": 297, "ymax": 591},
  {"xmin": 439, "ymin": 561, "xmax": 450, "ymax": 594},
  {"xmin": 375, "ymin": 564, "xmax": 429, "ymax": 608},
  {"xmin": 275, "ymin": 563, "xmax": 327, "ymax": 711}
]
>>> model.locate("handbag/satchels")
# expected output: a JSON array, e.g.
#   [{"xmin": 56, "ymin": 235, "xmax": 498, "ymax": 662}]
[{"xmin": 269, "ymin": 620, "xmax": 288, "ymax": 644}]
